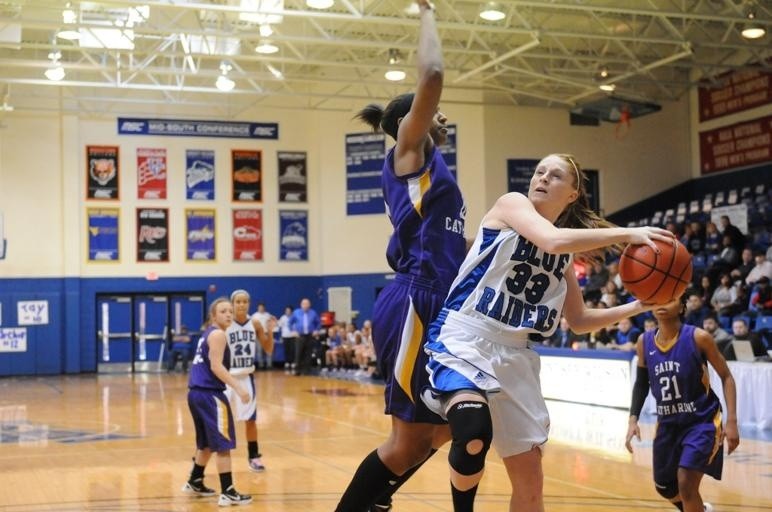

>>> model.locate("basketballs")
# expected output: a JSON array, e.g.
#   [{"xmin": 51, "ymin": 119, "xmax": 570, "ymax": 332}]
[{"xmin": 621, "ymin": 237, "xmax": 692, "ymax": 302}]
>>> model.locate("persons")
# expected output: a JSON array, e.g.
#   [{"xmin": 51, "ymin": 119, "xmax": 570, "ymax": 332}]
[
  {"xmin": 245, "ymin": 296, "xmax": 383, "ymax": 383},
  {"xmin": 522, "ymin": 210, "xmax": 772, "ymax": 360},
  {"xmin": 223, "ymin": 288, "xmax": 277, "ymax": 471},
  {"xmin": 419, "ymin": 151, "xmax": 691, "ymax": 512},
  {"xmin": 165, "ymin": 324, "xmax": 192, "ymax": 374},
  {"xmin": 182, "ymin": 296, "xmax": 253, "ymax": 507},
  {"xmin": 625, "ymin": 292, "xmax": 741, "ymax": 512},
  {"xmin": 335, "ymin": 2, "xmax": 469, "ymax": 512}
]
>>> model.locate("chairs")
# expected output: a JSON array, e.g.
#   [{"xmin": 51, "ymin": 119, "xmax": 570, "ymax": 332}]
[{"xmin": 628, "ymin": 184, "xmax": 772, "ymax": 346}]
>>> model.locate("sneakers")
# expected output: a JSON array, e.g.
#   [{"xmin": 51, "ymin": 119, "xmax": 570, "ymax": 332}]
[
  {"xmin": 219, "ymin": 488, "xmax": 253, "ymax": 507},
  {"xmin": 183, "ymin": 478, "xmax": 216, "ymax": 496},
  {"xmin": 249, "ymin": 456, "xmax": 265, "ymax": 472}
]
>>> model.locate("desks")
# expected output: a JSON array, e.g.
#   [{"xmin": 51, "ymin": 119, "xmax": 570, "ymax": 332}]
[{"xmin": 533, "ymin": 346, "xmax": 772, "ymax": 443}]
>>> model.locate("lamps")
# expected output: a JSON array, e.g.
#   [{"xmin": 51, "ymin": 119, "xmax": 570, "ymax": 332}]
[{"xmin": 740, "ymin": 3, "xmax": 766, "ymax": 39}]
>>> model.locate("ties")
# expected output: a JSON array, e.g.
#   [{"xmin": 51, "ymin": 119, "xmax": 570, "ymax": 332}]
[{"xmin": 303, "ymin": 312, "xmax": 308, "ymax": 335}]
